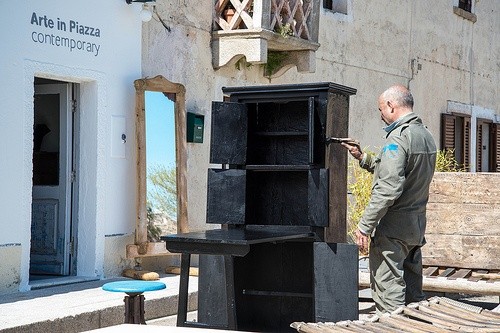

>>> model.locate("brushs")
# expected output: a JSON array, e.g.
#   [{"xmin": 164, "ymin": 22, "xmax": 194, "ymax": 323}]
[{"xmin": 327, "ymin": 137, "xmax": 356, "ymax": 145}]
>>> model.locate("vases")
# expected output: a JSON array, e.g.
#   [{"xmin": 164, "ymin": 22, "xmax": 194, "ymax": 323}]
[{"xmin": 224, "ymin": 9, "xmax": 243, "ymax": 29}]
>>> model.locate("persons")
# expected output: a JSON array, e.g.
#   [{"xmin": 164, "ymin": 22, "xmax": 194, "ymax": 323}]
[{"xmin": 341, "ymin": 84, "xmax": 437, "ymax": 317}]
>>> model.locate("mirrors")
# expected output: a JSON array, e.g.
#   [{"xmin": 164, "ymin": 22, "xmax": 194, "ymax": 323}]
[{"xmin": 125, "ymin": 75, "xmax": 198, "ymax": 280}]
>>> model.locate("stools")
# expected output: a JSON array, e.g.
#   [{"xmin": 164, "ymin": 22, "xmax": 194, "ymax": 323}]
[{"xmin": 103, "ymin": 281, "xmax": 166, "ymax": 324}]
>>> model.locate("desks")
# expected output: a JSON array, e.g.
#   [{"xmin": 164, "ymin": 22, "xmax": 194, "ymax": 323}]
[{"xmin": 160, "ymin": 231, "xmax": 312, "ymax": 330}]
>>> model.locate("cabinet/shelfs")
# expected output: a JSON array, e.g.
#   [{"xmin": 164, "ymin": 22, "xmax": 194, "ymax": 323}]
[{"xmin": 197, "ymin": 83, "xmax": 358, "ymax": 333}]
